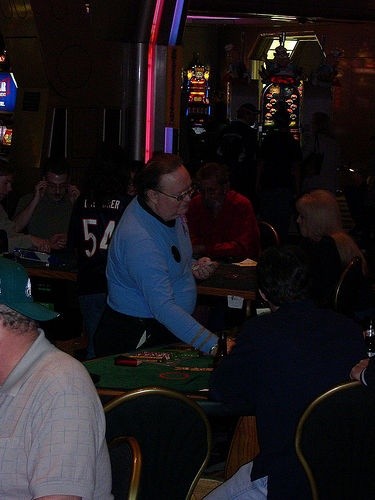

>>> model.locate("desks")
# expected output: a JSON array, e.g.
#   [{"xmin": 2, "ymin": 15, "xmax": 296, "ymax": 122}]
[
  {"xmin": 0, "ymin": 248, "xmax": 259, "ymax": 319},
  {"xmin": 82, "ymin": 330, "xmax": 253, "ymax": 413}
]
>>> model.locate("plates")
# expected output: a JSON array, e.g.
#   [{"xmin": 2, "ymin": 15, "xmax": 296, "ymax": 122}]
[{"xmin": 9, "ymin": 248, "xmax": 50, "ymax": 263}]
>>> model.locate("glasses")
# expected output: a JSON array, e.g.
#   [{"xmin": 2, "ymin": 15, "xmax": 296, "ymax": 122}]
[
  {"xmin": 153, "ymin": 187, "xmax": 196, "ymax": 201},
  {"xmin": 196, "ymin": 188, "xmax": 216, "ymax": 195},
  {"xmin": 46, "ymin": 183, "xmax": 67, "ymax": 189}
]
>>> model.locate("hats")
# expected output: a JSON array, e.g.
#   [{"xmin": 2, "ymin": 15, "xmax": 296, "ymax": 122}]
[{"xmin": 0, "ymin": 256, "xmax": 61, "ymax": 321}]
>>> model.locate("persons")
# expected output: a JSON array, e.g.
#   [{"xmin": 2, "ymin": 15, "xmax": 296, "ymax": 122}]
[
  {"xmin": 204, "ymin": 249, "xmax": 367, "ymax": 496},
  {"xmin": 0, "ymin": 258, "xmax": 112, "ymax": 496},
  {"xmin": 0, "ymin": 100, "xmax": 375, "ymax": 310},
  {"xmin": 347, "ymin": 355, "xmax": 374, "ymax": 388},
  {"xmin": 104, "ymin": 154, "xmax": 236, "ymax": 356}
]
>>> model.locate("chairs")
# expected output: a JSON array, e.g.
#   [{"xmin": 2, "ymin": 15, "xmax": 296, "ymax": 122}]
[
  {"xmin": 257, "ymin": 221, "xmax": 280, "ymax": 257},
  {"xmin": 334, "ymin": 256, "xmax": 362, "ymax": 313},
  {"xmin": 104, "ymin": 386, "xmax": 260, "ymax": 500},
  {"xmin": 295, "ymin": 381, "xmax": 375, "ymax": 500}
]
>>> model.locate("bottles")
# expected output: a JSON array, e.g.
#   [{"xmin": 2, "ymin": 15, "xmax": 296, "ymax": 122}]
[
  {"xmin": 211, "ymin": 331, "xmax": 227, "ymax": 374},
  {"xmin": 365, "ymin": 318, "xmax": 375, "ymax": 359}
]
